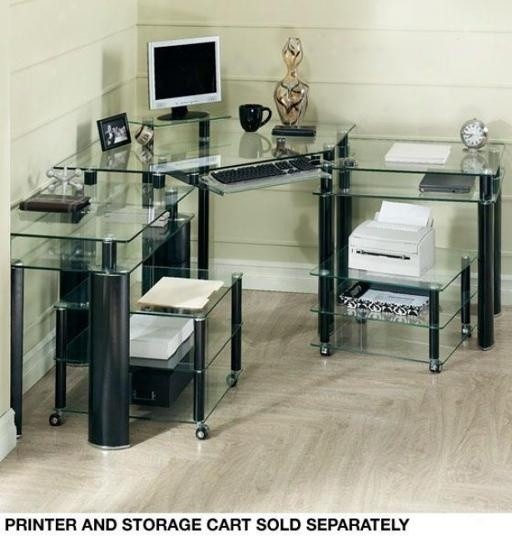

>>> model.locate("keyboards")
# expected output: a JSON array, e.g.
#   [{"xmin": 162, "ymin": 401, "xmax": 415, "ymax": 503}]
[{"xmin": 211, "ymin": 158, "xmax": 314, "ymax": 183}]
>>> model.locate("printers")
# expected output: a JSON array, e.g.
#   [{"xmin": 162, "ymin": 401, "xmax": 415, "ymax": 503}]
[{"xmin": 348, "ymin": 212, "xmax": 434, "ymax": 277}]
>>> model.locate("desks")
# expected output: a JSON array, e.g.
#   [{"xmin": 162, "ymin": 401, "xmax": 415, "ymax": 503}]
[{"xmin": 54, "ymin": 113, "xmax": 362, "ymax": 369}]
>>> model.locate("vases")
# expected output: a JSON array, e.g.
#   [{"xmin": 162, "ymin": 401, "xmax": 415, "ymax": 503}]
[{"xmin": 274, "ymin": 36, "xmax": 310, "ymax": 128}]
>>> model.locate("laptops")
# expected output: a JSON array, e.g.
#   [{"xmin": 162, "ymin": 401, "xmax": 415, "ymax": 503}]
[{"xmin": 419, "ymin": 174, "xmax": 475, "ymax": 193}]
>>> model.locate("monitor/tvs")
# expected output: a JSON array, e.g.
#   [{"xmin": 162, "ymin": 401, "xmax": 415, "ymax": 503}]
[{"xmin": 147, "ymin": 36, "xmax": 222, "ymax": 120}]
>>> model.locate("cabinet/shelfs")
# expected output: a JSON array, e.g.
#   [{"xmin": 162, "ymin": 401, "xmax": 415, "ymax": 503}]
[
  {"xmin": 310, "ymin": 136, "xmax": 510, "ymax": 352},
  {"xmin": 51, "ymin": 260, "xmax": 251, "ymax": 440},
  {"xmin": 308, "ymin": 244, "xmax": 480, "ymax": 375},
  {"xmin": 11, "ymin": 182, "xmax": 201, "ymax": 449}
]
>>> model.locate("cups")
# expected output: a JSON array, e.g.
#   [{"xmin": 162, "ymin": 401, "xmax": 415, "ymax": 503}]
[
  {"xmin": 238, "ymin": 131, "xmax": 272, "ymax": 160},
  {"xmin": 239, "ymin": 104, "xmax": 272, "ymax": 133}
]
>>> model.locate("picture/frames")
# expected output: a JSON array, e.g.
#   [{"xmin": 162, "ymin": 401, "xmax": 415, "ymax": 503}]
[
  {"xmin": 99, "ymin": 147, "xmax": 130, "ymax": 170},
  {"xmin": 96, "ymin": 113, "xmax": 131, "ymax": 149}
]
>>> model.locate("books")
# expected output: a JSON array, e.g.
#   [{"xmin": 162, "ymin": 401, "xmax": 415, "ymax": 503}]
[{"xmin": 20, "ymin": 195, "xmax": 91, "ymax": 214}]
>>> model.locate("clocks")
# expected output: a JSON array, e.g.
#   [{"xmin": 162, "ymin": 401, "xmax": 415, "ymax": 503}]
[
  {"xmin": 459, "ymin": 117, "xmax": 489, "ymax": 152},
  {"xmin": 459, "ymin": 154, "xmax": 488, "ymax": 175}
]
